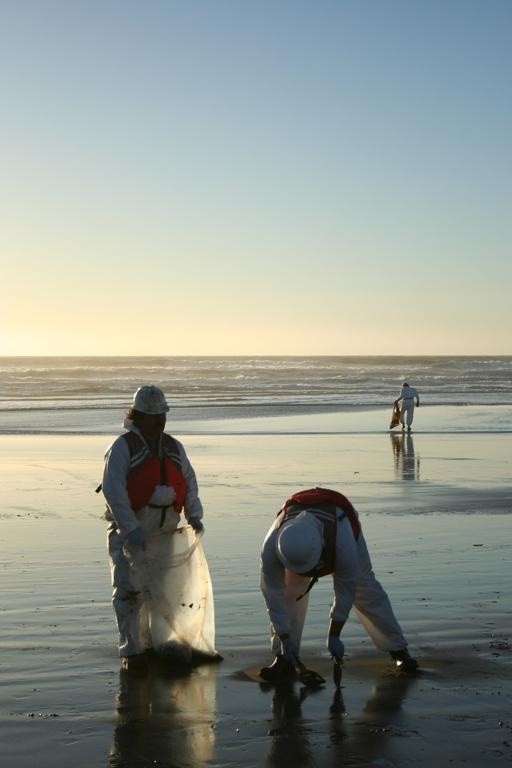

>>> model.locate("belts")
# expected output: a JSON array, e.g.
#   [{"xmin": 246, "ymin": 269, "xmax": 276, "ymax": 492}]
[{"xmin": 403, "ymin": 397, "xmax": 414, "ymax": 400}]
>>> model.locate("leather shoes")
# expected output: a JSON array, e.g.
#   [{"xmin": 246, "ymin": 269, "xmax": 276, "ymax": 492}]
[
  {"xmin": 390, "ymin": 649, "xmax": 418, "ymax": 673},
  {"xmin": 259, "ymin": 653, "xmax": 297, "ymax": 682}
]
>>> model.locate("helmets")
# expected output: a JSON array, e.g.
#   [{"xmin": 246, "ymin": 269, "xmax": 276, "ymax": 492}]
[
  {"xmin": 275, "ymin": 517, "xmax": 325, "ymax": 575},
  {"xmin": 131, "ymin": 385, "xmax": 170, "ymax": 415}
]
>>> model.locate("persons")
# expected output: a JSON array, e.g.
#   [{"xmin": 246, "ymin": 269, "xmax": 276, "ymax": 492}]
[
  {"xmin": 101, "ymin": 384, "xmax": 204, "ymax": 670},
  {"xmin": 394, "ymin": 382, "xmax": 419, "ymax": 431},
  {"xmin": 259, "ymin": 487, "xmax": 418, "ymax": 681}
]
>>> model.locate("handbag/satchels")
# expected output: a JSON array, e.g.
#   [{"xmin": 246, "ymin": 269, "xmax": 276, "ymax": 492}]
[{"xmin": 390, "ymin": 405, "xmax": 401, "ymax": 429}]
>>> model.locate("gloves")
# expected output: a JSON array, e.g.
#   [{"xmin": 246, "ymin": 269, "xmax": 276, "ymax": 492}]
[
  {"xmin": 279, "ymin": 637, "xmax": 299, "ymax": 665},
  {"xmin": 127, "ymin": 528, "xmax": 147, "ymax": 548},
  {"xmin": 326, "ymin": 635, "xmax": 345, "ymax": 666},
  {"xmin": 187, "ymin": 516, "xmax": 203, "ymax": 535}
]
